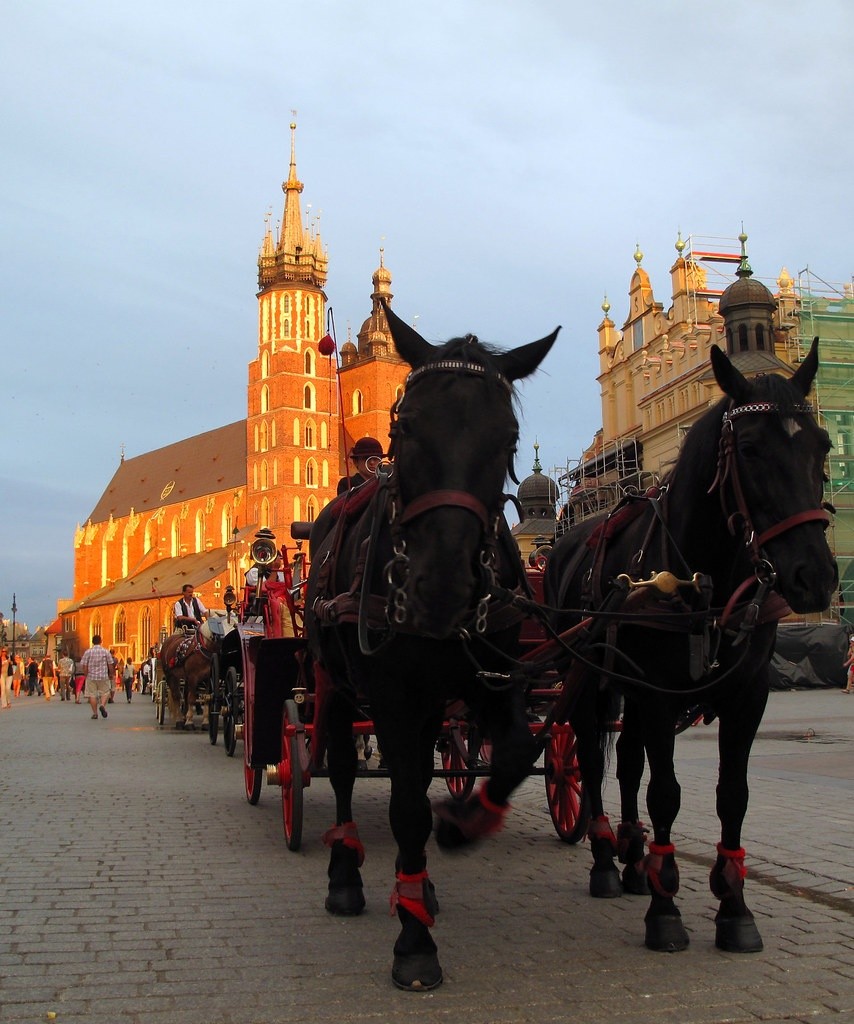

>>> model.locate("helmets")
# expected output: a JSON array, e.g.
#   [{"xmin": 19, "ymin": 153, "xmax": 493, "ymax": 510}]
[{"xmin": 350, "ymin": 437, "xmax": 386, "ymax": 457}]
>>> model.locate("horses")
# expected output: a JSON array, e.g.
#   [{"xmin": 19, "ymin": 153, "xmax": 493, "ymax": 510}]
[{"xmin": 302, "ymin": 293, "xmax": 840, "ymax": 991}]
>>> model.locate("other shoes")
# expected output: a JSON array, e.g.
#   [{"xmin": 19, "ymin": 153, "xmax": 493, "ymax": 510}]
[
  {"xmin": 108, "ymin": 697, "xmax": 114, "ymax": 703},
  {"xmin": 67, "ymin": 698, "xmax": 70, "ymax": 700},
  {"xmin": 76, "ymin": 700, "xmax": 81, "ymax": 704},
  {"xmin": 99, "ymin": 705, "xmax": 107, "ymax": 718},
  {"xmin": 91, "ymin": 715, "xmax": 97, "ymax": 719},
  {"xmin": 61, "ymin": 698, "xmax": 64, "ymax": 701}
]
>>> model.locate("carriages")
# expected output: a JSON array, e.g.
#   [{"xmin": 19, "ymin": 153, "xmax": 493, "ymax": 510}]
[
  {"xmin": 152, "ymin": 602, "xmax": 248, "ymax": 735},
  {"xmin": 225, "ymin": 295, "xmax": 842, "ymax": 993}
]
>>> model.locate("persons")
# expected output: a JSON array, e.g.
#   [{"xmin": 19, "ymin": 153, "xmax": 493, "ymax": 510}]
[
  {"xmin": 79, "ymin": 635, "xmax": 114, "ymax": 720},
  {"xmin": 107, "ymin": 649, "xmax": 141, "ymax": 704},
  {"xmin": 336, "ymin": 436, "xmax": 389, "ymax": 498},
  {"xmin": 243, "ymin": 548, "xmax": 284, "ymax": 595},
  {"xmin": 0, "ymin": 645, "xmax": 86, "ymax": 710},
  {"xmin": 174, "ymin": 584, "xmax": 213, "ymax": 636}
]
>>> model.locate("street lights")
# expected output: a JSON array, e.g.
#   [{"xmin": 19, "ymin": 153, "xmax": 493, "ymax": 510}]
[{"xmin": 11, "ymin": 592, "xmax": 18, "ymax": 665}]
